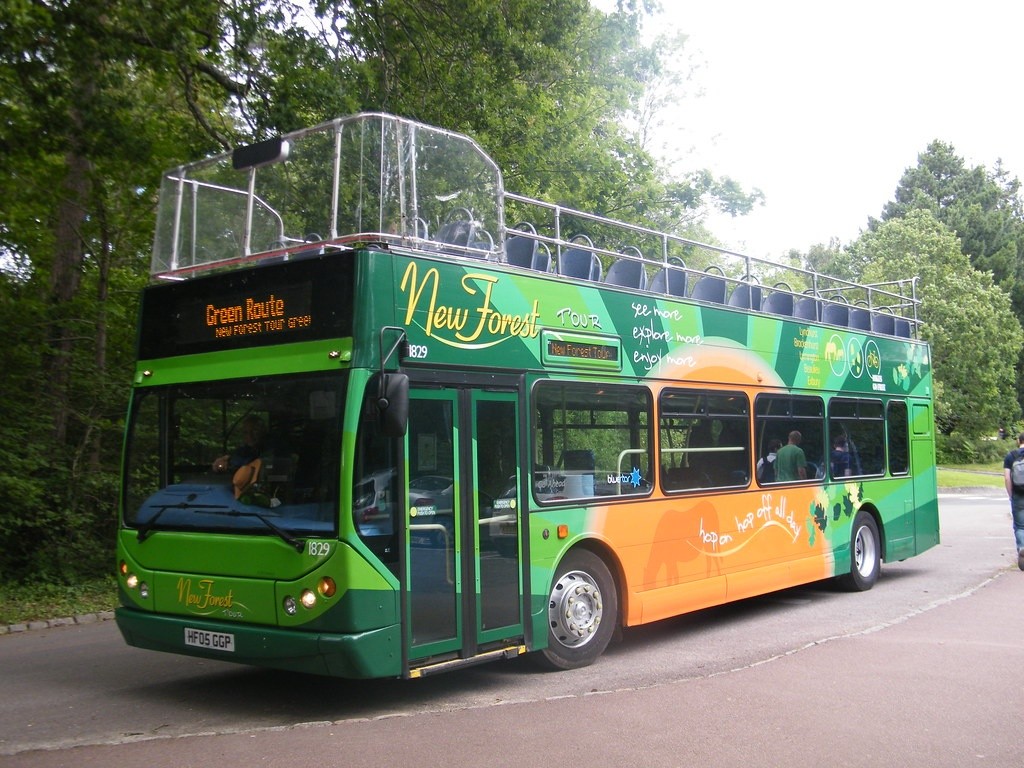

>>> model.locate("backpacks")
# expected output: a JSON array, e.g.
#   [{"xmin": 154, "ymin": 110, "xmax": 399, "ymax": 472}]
[
  {"xmin": 761, "ymin": 457, "xmax": 776, "ymax": 482},
  {"xmin": 1011, "ymin": 450, "xmax": 1024, "ymax": 493},
  {"xmin": 814, "ymin": 461, "xmax": 834, "ymax": 478}
]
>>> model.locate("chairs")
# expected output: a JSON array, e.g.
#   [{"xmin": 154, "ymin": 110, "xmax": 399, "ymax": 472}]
[
  {"xmin": 660, "ymin": 457, "xmax": 886, "ymax": 496},
  {"xmin": 258, "ymin": 208, "xmax": 911, "ymax": 340}
]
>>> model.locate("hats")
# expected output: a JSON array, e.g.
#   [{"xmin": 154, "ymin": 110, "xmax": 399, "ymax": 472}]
[{"xmin": 232, "ymin": 458, "xmax": 262, "ymax": 501}]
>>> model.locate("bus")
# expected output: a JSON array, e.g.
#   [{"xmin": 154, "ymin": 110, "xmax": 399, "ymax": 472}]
[{"xmin": 115, "ymin": 114, "xmax": 940, "ymax": 683}]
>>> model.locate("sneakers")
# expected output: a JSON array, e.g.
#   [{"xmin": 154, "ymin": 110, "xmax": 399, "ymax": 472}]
[{"xmin": 1017, "ymin": 547, "xmax": 1023, "ymax": 571}]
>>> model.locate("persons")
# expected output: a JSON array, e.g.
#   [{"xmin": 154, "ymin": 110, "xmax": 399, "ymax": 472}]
[
  {"xmin": 1004, "ymin": 433, "xmax": 1024, "ymax": 571},
  {"xmin": 758, "ymin": 430, "xmax": 856, "ymax": 483},
  {"xmin": 213, "ymin": 417, "xmax": 273, "ymax": 483}
]
